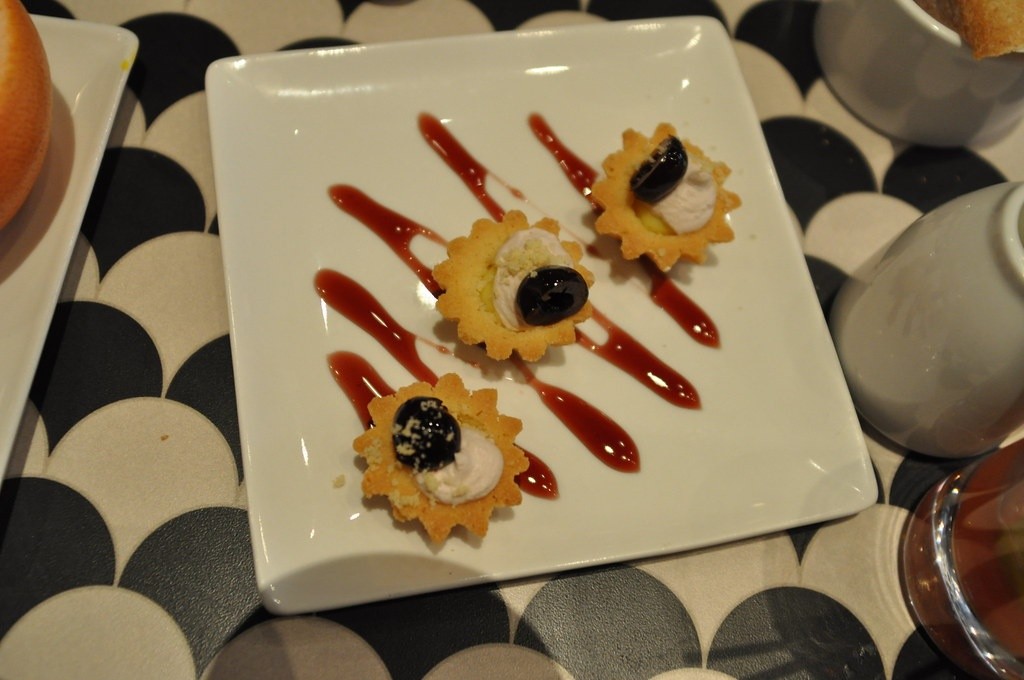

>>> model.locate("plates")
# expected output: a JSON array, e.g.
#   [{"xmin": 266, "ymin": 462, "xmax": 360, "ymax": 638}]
[
  {"xmin": 204, "ymin": 15, "xmax": 879, "ymax": 615},
  {"xmin": 0, "ymin": 13, "xmax": 140, "ymax": 484}
]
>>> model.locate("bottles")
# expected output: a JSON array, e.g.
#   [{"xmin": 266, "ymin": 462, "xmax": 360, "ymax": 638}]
[{"xmin": 830, "ymin": 182, "xmax": 1024, "ymax": 459}]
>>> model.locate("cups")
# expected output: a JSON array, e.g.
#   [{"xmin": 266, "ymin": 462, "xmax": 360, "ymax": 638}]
[
  {"xmin": 813, "ymin": 0, "xmax": 1024, "ymax": 147},
  {"xmin": 904, "ymin": 437, "xmax": 1024, "ymax": 680}
]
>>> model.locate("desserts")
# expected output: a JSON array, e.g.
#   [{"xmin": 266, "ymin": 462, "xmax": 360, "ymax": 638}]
[
  {"xmin": 353, "ymin": 373, "xmax": 531, "ymax": 546},
  {"xmin": 432, "ymin": 209, "xmax": 597, "ymax": 363},
  {"xmin": 592, "ymin": 122, "xmax": 743, "ymax": 273}
]
